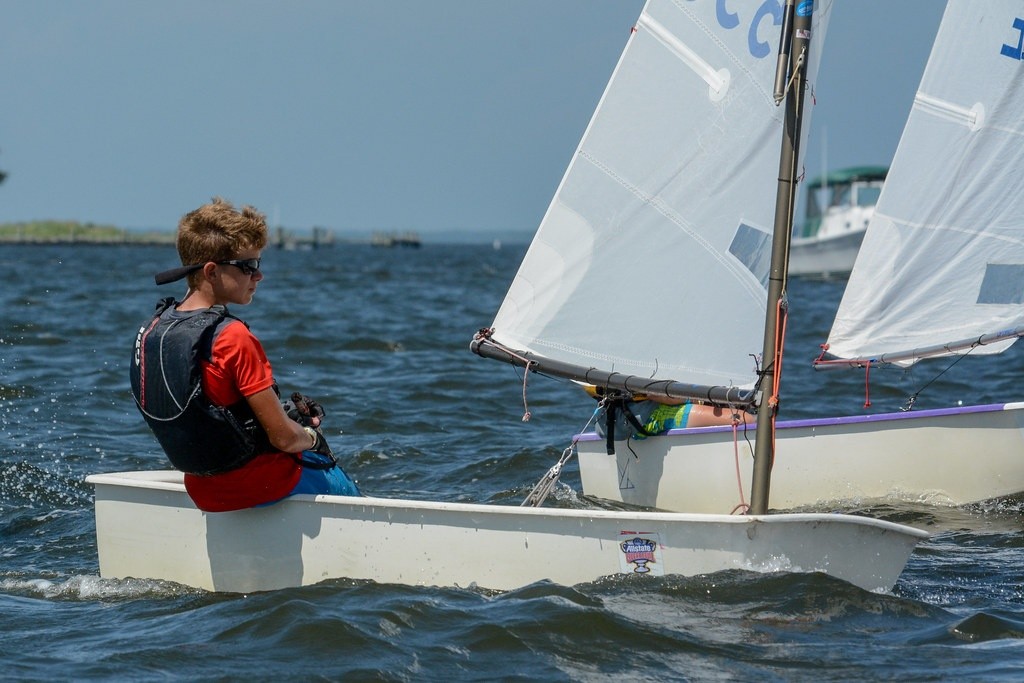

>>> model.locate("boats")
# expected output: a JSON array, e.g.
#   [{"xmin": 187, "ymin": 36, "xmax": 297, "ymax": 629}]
[{"xmin": 787, "ymin": 164, "xmax": 897, "ymax": 285}]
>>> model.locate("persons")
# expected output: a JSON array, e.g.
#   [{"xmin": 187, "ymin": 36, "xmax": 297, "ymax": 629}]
[
  {"xmin": 129, "ymin": 196, "xmax": 363, "ymax": 514},
  {"xmin": 583, "ymin": 381, "xmax": 758, "ymax": 442}
]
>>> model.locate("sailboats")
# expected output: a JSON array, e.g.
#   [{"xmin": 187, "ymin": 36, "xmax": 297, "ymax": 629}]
[
  {"xmin": 568, "ymin": 0, "xmax": 1024, "ymax": 520},
  {"xmin": 85, "ymin": 2, "xmax": 933, "ymax": 609}
]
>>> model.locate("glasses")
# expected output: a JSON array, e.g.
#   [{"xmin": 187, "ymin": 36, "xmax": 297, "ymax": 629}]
[{"xmin": 153, "ymin": 258, "xmax": 260, "ymax": 286}]
